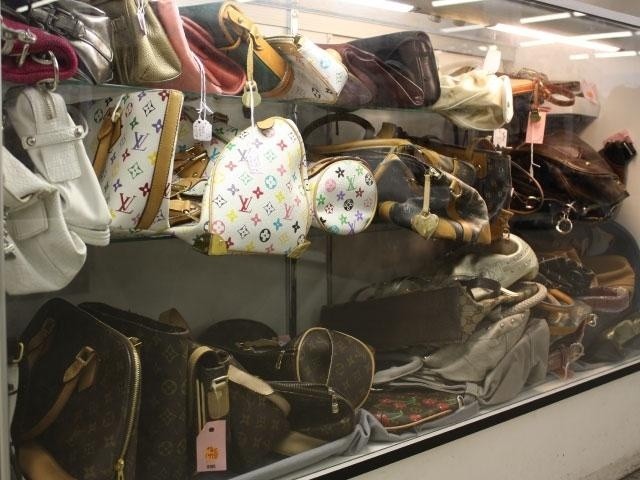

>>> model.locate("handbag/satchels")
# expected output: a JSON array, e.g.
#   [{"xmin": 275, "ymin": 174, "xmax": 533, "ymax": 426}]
[
  {"xmin": 1, "ymin": 0, "xmax": 636, "ymax": 298},
  {"xmin": 7, "ymin": 297, "xmax": 374, "ymax": 479},
  {"xmin": 318, "ymin": 232, "xmax": 639, "ymax": 431}
]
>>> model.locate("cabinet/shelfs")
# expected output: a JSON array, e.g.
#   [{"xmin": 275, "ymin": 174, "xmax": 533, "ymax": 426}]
[{"xmin": 0, "ymin": 2, "xmax": 640, "ymax": 479}]
